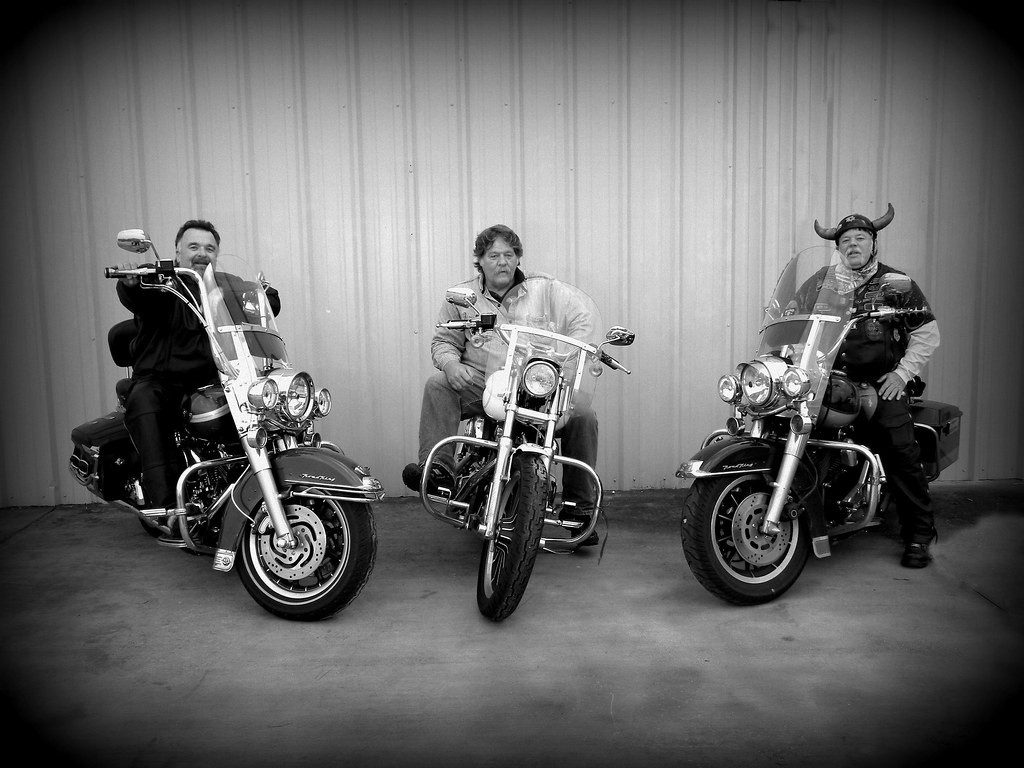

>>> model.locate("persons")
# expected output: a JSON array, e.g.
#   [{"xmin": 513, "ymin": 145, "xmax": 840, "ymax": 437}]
[
  {"xmin": 117, "ymin": 220, "xmax": 281, "ymax": 509},
  {"xmin": 764, "ymin": 203, "xmax": 941, "ymax": 568},
  {"xmin": 402, "ymin": 225, "xmax": 600, "ymax": 546}
]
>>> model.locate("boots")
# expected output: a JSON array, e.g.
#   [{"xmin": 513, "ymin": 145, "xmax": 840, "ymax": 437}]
[{"xmin": 144, "ymin": 468, "xmax": 185, "ymax": 536}]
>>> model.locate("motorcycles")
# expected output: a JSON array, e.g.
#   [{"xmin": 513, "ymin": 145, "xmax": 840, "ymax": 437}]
[
  {"xmin": 419, "ymin": 276, "xmax": 637, "ymax": 623},
  {"xmin": 66, "ymin": 228, "xmax": 386, "ymax": 624},
  {"xmin": 671, "ymin": 245, "xmax": 962, "ymax": 607}
]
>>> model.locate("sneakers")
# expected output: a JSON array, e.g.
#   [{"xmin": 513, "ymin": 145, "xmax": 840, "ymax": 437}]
[
  {"xmin": 559, "ymin": 509, "xmax": 599, "ymax": 546},
  {"xmin": 402, "ymin": 463, "xmax": 458, "ymax": 500},
  {"xmin": 900, "ymin": 542, "xmax": 929, "ymax": 569}
]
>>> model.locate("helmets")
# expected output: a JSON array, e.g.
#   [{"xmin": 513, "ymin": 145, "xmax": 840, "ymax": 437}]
[{"xmin": 814, "ymin": 203, "xmax": 895, "ymax": 246}]
